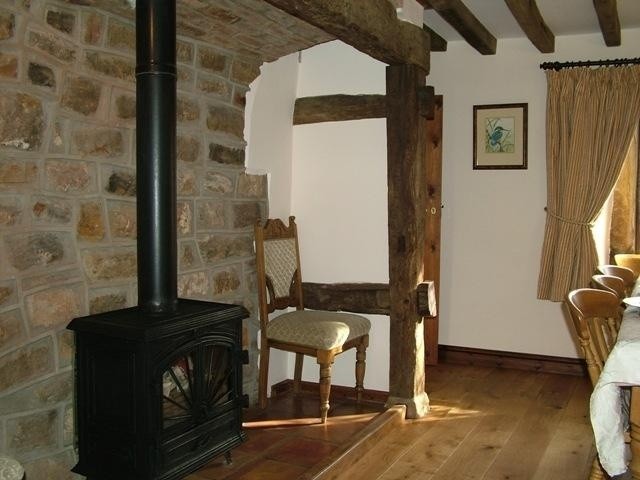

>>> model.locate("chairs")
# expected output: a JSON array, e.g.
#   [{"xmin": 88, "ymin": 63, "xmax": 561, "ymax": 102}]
[
  {"xmin": 613, "ymin": 253, "xmax": 640, "ymax": 282},
  {"xmin": 254, "ymin": 216, "xmax": 371, "ymax": 424},
  {"xmin": 596, "ymin": 264, "xmax": 634, "ymax": 296},
  {"xmin": 565, "ymin": 289, "xmax": 631, "ymax": 480},
  {"xmin": 590, "ymin": 274, "xmax": 625, "ymax": 317}
]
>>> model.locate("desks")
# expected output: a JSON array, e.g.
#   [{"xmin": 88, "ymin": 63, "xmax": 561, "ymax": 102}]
[{"xmin": 581, "ymin": 272, "xmax": 640, "ymax": 480}]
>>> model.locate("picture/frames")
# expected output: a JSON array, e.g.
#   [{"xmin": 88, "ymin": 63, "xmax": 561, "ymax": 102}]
[{"xmin": 473, "ymin": 103, "xmax": 527, "ymax": 170}]
